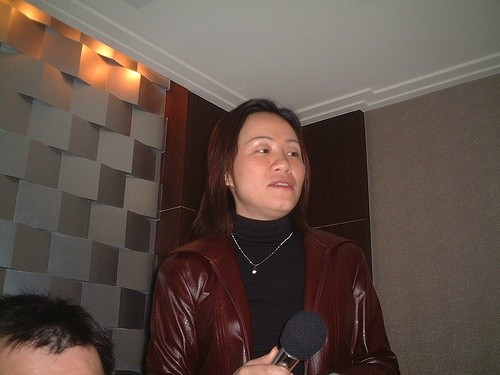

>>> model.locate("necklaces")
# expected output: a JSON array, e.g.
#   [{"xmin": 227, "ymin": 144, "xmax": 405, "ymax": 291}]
[{"xmin": 231, "ymin": 230, "xmax": 293, "ymax": 274}]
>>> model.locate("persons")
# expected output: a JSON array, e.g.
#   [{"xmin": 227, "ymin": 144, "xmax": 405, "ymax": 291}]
[
  {"xmin": 0, "ymin": 282, "xmax": 117, "ymax": 374},
  {"xmin": 142, "ymin": 96, "xmax": 407, "ymax": 375}
]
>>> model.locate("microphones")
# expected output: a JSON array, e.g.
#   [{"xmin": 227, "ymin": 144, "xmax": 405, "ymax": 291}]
[{"xmin": 271, "ymin": 310, "xmax": 328, "ymax": 371}]
